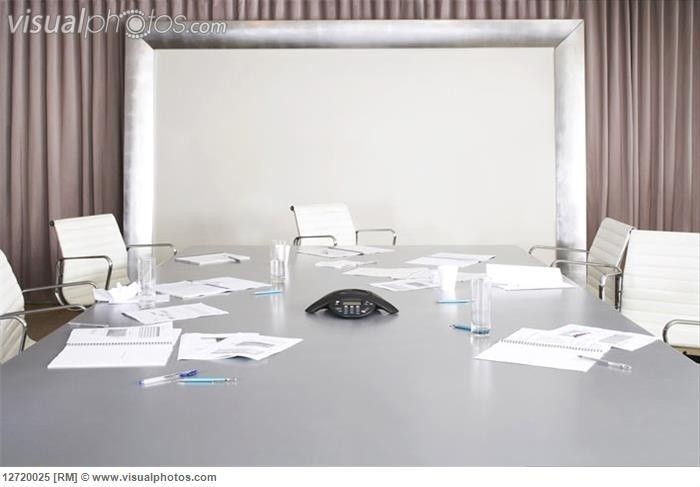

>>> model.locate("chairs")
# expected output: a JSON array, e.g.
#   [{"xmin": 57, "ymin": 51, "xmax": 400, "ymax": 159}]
[
  {"xmin": 598, "ymin": 227, "xmax": 700, "ymax": 359},
  {"xmin": 288, "ymin": 198, "xmax": 398, "ymax": 249},
  {"xmin": 528, "ymin": 216, "xmax": 635, "ymax": 314},
  {"xmin": 0, "ymin": 245, "xmax": 104, "ymax": 367},
  {"xmin": 47, "ymin": 210, "xmax": 179, "ymax": 313}
]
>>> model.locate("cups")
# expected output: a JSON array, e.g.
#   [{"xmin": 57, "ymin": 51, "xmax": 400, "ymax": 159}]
[
  {"xmin": 276, "ymin": 244, "xmax": 291, "ymax": 260},
  {"xmin": 469, "ymin": 275, "xmax": 493, "ymax": 336},
  {"xmin": 268, "ymin": 238, "xmax": 288, "ymax": 284},
  {"xmin": 437, "ymin": 263, "xmax": 460, "ymax": 292},
  {"xmin": 136, "ymin": 256, "xmax": 157, "ymax": 309}
]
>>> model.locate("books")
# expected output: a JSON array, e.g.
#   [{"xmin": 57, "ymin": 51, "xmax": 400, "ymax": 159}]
[
  {"xmin": 153, "ymin": 275, "xmax": 272, "ymax": 297},
  {"xmin": 485, "ymin": 262, "xmax": 571, "ymax": 290},
  {"xmin": 298, "ymin": 244, "xmax": 391, "ymax": 259},
  {"xmin": 408, "ymin": 251, "xmax": 496, "ymax": 270},
  {"xmin": 175, "ymin": 252, "xmax": 248, "ymax": 266},
  {"xmin": 477, "ymin": 326, "xmax": 612, "ymax": 374},
  {"xmin": 45, "ymin": 326, "xmax": 182, "ymax": 369}
]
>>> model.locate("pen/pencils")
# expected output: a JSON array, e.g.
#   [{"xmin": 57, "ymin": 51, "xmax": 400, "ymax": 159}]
[
  {"xmin": 68, "ymin": 322, "xmax": 109, "ymax": 327},
  {"xmin": 175, "ymin": 378, "xmax": 238, "ymax": 382},
  {"xmin": 436, "ymin": 300, "xmax": 470, "ymax": 303},
  {"xmin": 578, "ymin": 355, "xmax": 632, "ymax": 370},
  {"xmin": 138, "ymin": 369, "xmax": 197, "ymax": 386},
  {"xmin": 453, "ymin": 324, "xmax": 489, "ymax": 334},
  {"xmin": 251, "ymin": 291, "xmax": 280, "ymax": 295}
]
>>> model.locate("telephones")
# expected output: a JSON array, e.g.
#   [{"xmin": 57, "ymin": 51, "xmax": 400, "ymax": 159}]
[{"xmin": 305, "ymin": 289, "xmax": 400, "ymax": 319}]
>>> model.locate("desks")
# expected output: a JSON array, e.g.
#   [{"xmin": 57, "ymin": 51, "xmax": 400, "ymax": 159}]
[{"xmin": 1, "ymin": 245, "xmax": 700, "ymax": 468}]
